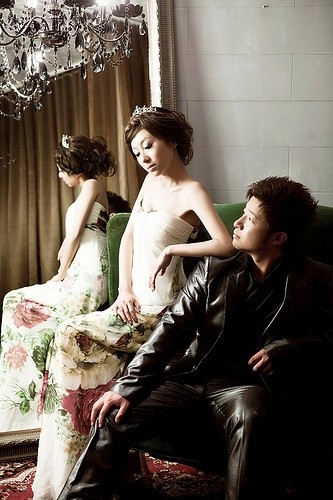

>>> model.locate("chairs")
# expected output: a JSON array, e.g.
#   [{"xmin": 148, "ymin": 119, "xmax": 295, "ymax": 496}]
[
  {"xmin": 108, "ymin": 211, "xmax": 129, "ymax": 305},
  {"xmin": 128, "ymin": 202, "xmax": 333, "ymax": 494}
]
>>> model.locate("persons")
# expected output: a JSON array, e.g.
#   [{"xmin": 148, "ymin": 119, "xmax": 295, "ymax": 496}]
[
  {"xmin": 0, "ymin": 134, "xmax": 133, "ymax": 433},
  {"xmin": 59, "ymin": 177, "xmax": 333, "ymax": 500},
  {"xmin": 31, "ymin": 105, "xmax": 237, "ymax": 500}
]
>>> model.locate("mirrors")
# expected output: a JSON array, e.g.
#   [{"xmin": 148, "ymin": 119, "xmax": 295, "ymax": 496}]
[{"xmin": 0, "ymin": 0, "xmax": 177, "ymax": 296}]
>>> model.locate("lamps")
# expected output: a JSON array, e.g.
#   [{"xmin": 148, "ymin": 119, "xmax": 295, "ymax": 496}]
[
  {"xmin": 0, "ymin": 0, "xmax": 146, "ymax": 94},
  {"xmin": 0, "ymin": 0, "xmax": 52, "ymax": 120}
]
